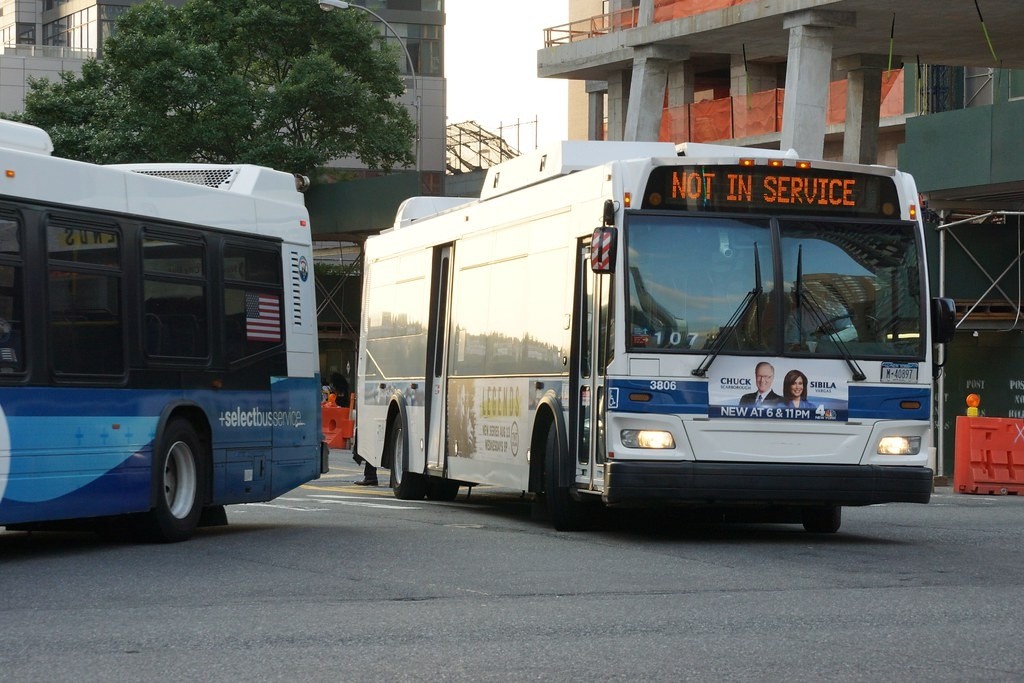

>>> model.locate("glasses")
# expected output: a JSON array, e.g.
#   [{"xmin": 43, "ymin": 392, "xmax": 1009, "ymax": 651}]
[{"xmin": 757, "ymin": 375, "xmax": 772, "ymax": 381}]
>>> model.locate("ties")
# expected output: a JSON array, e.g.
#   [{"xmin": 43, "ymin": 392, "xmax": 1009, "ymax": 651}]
[{"xmin": 755, "ymin": 395, "xmax": 763, "ymax": 407}]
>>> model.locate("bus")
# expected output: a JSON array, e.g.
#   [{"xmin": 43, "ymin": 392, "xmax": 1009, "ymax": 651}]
[
  {"xmin": 0, "ymin": 116, "xmax": 329, "ymax": 542},
  {"xmin": 354, "ymin": 139, "xmax": 956, "ymax": 535}
]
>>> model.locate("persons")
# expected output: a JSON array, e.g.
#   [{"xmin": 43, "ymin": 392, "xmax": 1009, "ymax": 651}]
[
  {"xmin": 785, "ymin": 279, "xmax": 858, "ymax": 347},
  {"xmin": 774, "ymin": 370, "xmax": 815, "ymax": 409},
  {"xmin": 738, "ymin": 361, "xmax": 783, "ymax": 408},
  {"xmin": 330, "ymin": 373, "xmax": 350, "ymax": 407},
  {"xmin": 321, "ymin": 377, "xmax": 330, "ymax": 403}
]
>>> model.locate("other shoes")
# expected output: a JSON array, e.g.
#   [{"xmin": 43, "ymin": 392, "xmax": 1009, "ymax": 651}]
[{"xmin": 354, "ymin": 480, "xmax": 378, "ymax": 486}]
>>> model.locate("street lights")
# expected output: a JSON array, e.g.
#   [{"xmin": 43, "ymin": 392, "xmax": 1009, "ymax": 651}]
[{"xmin": 317, "ymin": 0, "xmax": 421, "ymax": 194}]
